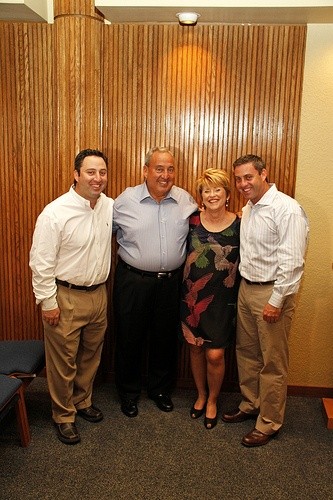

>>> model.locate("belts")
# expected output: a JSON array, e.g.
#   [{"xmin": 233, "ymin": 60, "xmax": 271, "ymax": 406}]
[
  {"xmin": 55, "ymin": 279, "xmax": 101, "ymax": 292},
  {"xmin": 244, "ymin": 278, "xmax": 277, "ymax": 286},
  {"xmin": 118, "ymin": 256, "xmax": 180, "ymax": 279}
]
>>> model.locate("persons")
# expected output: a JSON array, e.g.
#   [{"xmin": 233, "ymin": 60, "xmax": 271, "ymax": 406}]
[
  {"xmin": 110, "ymin": 144, "xmax": 199, "ymax": 416},
  {"xmin": 181, "ymin": 167, "xmax": 241, "ymax": 431},
  {"xmin": 28, "ymin": 148, "xmax": 114, "ymax": 443},
  {"xmin": 220, "ymin": 154, "xmax": 309, "ymax": 447}
]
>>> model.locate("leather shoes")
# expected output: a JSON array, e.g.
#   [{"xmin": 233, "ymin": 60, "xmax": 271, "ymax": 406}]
[
  {"xmin": 117, "ymin": 388, "xmax": 138, "ymax": 417},
  {"xmin": 75, "ymin": 404, "xmax": 103, "ymax": 422},
  {"xmin": 146, "ymin": 389, "xmax": 173, "ymax": 412},
  {"xmin": 190, "ymin": 398, "xmax": 207, "ymax": 418},
  {"xmin": 241, "ymin": 428, "xmax": 279, "ymax": 447},
  {"xmin": 220, "ymin": 407, "xmax": 255, "ymax": 423},
  {"xmin": 54, "ymin": 421, "xmax": 80, "ymax": 444},
  {"xmin": 204, "ymin": 403, "xmax": 217, "ymax": 429}
]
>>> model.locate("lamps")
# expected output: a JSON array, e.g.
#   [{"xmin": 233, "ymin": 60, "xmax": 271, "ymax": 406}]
[{"xmin": 177, "ymin": 13, "xmax": 197, "ymax": 24}]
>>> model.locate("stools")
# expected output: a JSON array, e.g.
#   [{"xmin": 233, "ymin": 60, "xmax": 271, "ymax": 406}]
[{"xmin": 0, "ymin": 339, "xmax": 47, "ymax": 445}]
